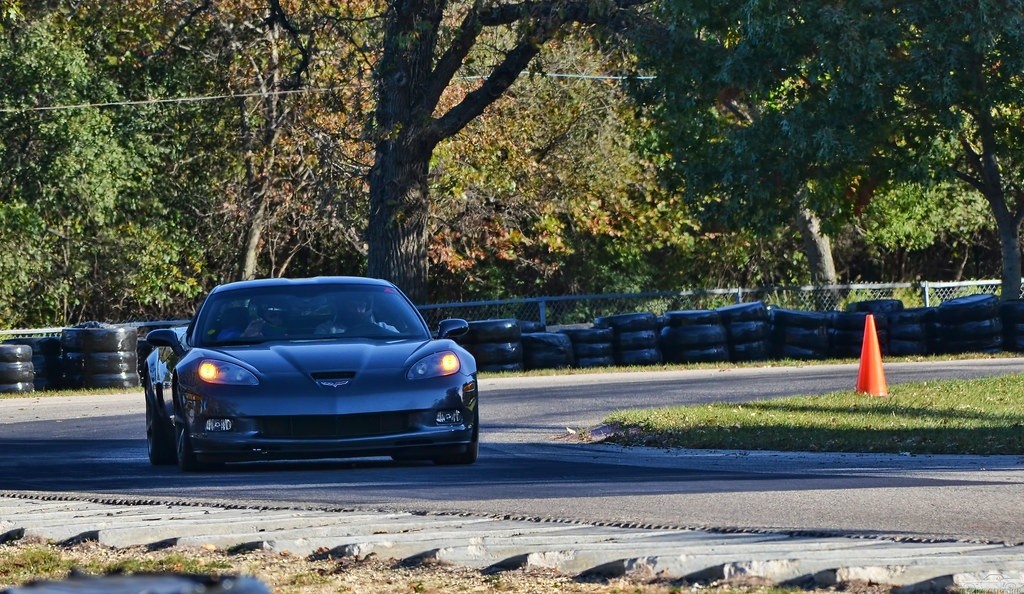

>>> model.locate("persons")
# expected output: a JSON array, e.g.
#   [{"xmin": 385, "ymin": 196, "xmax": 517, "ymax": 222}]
[
  {"xmin": 314, "ymin": 288, "xmax": 400, "ymax": 337},
  {"xmin": 216, "ymin": 291, "xmax": 295, "ymax": 342}
]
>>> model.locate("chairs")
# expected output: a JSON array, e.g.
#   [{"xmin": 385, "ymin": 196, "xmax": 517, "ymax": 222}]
[{"xmin": 220, "ymin": 307, "xmax": 249, "ymax": 330}]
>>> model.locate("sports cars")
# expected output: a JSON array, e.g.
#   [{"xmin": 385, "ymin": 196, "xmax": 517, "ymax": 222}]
[{"xmin": 143, "ymin": 276, "xmax": 479, "ymax": 473}]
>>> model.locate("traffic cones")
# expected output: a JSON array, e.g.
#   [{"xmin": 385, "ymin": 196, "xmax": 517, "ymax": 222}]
[{"xmin": 855, "ymin": 314, "xmax": 889, "ymax": 397}]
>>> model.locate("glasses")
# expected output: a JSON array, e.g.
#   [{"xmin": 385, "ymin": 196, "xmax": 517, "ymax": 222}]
[{"xmin": 345, "ymin": 301, "xmax": 367, "ymax": 311}]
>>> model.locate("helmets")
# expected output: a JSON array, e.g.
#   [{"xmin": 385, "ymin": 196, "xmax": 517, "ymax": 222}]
[
  {"xmin": 318, "ymin": 289, "xmax": 374, "ymax": 324},
  {"xmin": 247, "ymin": 293, "xmax": 290, "ymax": 338}
]
